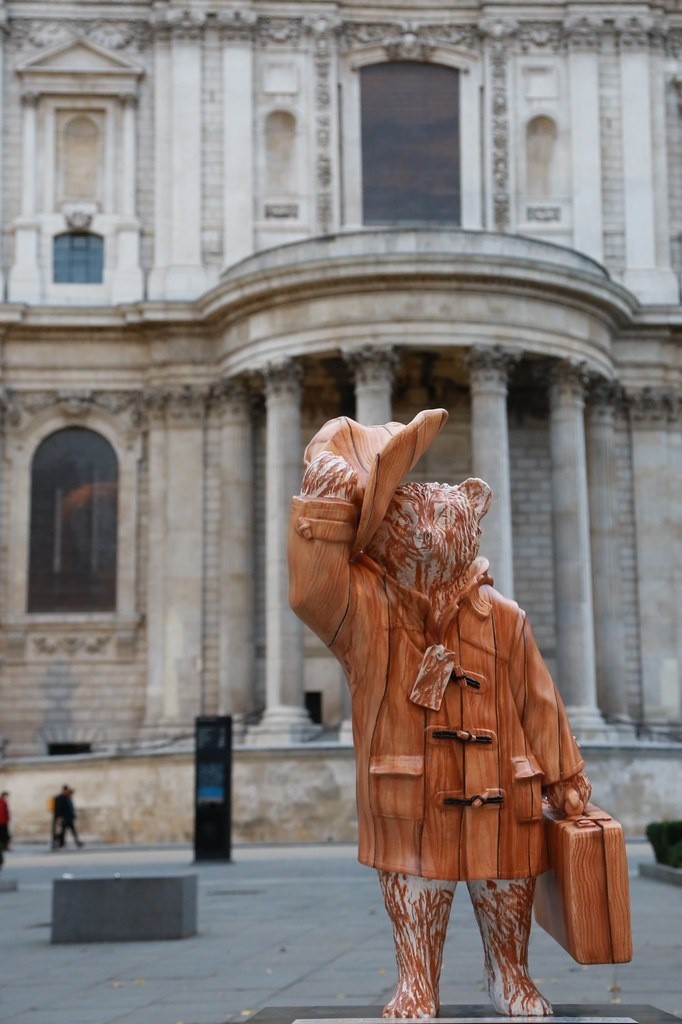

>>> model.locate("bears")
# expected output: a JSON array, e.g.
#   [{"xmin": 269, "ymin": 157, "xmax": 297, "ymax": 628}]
[{"xmin": 302, "ymin": 454, "xmax": 594, "ymax": 1024}]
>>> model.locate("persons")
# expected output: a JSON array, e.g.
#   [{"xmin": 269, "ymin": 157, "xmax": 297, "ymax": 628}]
[
  {"xmin": 57, "ymin": 789, "xmax": 84, "ymax": 849},
  {"xmin": 51, "ymin": 784, "xmax": 68, "ymax": 849},
  {"xmin": 0, "ymin": 792, "xmax": 12, "ymax": 851}
]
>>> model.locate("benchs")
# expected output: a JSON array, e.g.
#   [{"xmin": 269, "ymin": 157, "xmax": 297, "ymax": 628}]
[{"xmin": 51, "ymin": 875, "xmax": 199, "ymax": 945}]
